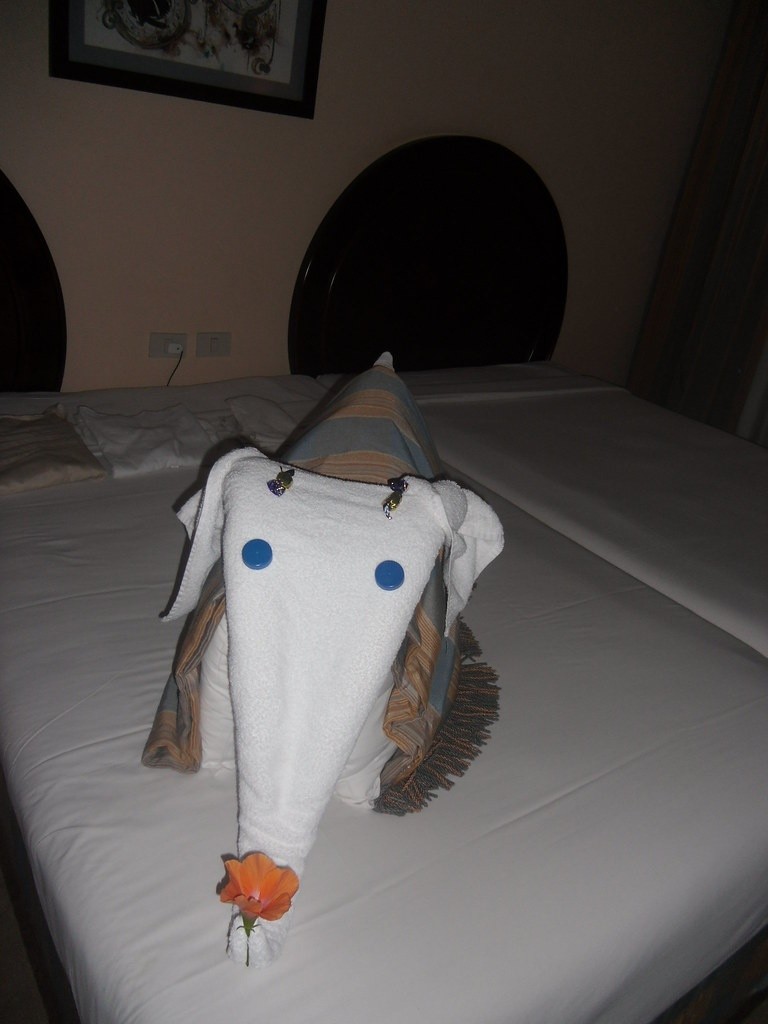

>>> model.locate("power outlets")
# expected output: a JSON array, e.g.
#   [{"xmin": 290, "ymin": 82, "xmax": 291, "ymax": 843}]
[
  {"xmin": 196, "ymin": 332, "xmax": 232, "ymax": 358},
  {"xmin": 148, "ymin": 332, "xmax": 188, "ymax": 359}
]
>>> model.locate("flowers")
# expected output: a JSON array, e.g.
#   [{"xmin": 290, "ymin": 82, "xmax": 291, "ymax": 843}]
[{"xmin": 219, "ymin": 850, "xmax": 299, "ymax": 968}]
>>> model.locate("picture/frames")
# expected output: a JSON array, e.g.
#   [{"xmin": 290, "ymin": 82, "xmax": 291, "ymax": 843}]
[{"xmin": 48, "ymin": 0, "xmax": 327, "ymax": 120}]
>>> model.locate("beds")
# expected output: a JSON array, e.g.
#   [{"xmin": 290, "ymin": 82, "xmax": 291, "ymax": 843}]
[
  {"xmin": 0, "ymin": 167, "xmax": 768, "ymax": 1024},
  {"xmin": 287, "ymin": 135, "xmax": 768, "ymax": 661}
]
